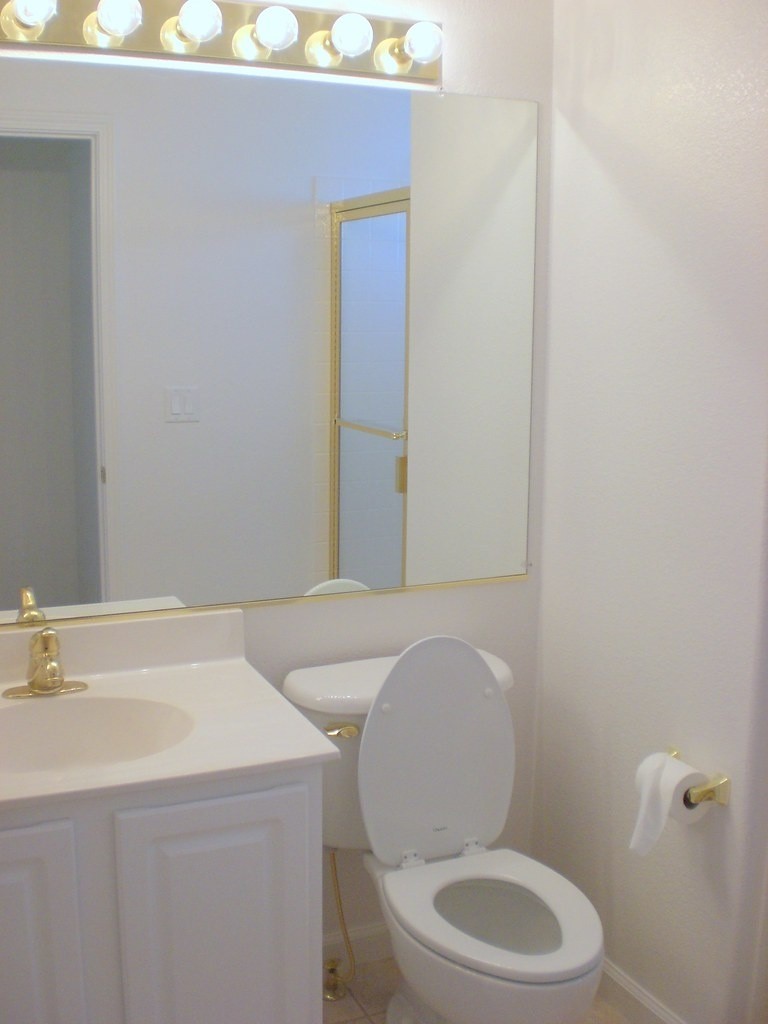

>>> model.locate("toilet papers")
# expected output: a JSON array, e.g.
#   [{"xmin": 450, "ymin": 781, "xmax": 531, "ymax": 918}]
[{"xmin": 629, "ymin": 752, "xmax": 715, "ymax": 856}]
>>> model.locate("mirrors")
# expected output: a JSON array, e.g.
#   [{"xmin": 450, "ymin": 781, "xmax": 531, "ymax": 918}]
[{"xmin": 0, "ymin": 48, "xmax": 544, "ymax": 632}]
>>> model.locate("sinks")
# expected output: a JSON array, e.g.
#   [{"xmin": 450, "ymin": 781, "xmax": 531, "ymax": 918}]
[{"xmin": 0, "ymin": 688, "xmax": 197, "ymax": 780}]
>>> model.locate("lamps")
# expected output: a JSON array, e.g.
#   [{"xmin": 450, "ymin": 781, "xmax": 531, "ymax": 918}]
[
  {"xmin": 0, "ymin": 0, "xmax": 58, "ymax": 42},
  {"xmin": 159, "ymin": 0, "xmax": 224, "ymax": 54},
  {"xmin": 373, "ymin": 21, "xmax": 443, "ymax": 76},
  {"xmin": 82, "ymin": 0, "xmax": 142, "ymax": 47},
  {"xmin": 304, "ymin": 12, "xmax": 374, "ymax": 68},
  {"xmin": 231, "ymin": 6, "xmax": 298, "ymax": 62}
]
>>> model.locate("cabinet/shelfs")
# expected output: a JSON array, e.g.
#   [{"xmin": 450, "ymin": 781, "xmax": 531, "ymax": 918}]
[{"xmin": 0, "ymin": 764, "xmax": 324, "ymax": 1024}]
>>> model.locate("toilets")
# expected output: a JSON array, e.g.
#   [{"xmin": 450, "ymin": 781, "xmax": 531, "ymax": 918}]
[{"xmin": 285, "ymin": 631, "xmax": 609, "ymax": 1024}]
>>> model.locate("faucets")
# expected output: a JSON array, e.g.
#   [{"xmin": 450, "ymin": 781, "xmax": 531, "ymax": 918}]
[
  {"xmin": 16, "ymin": 586, "xmax": 46, "ymax": 624},
  {"xmin": 26, "ymin": 628, "xmax": 64, "ymax": 694}
]
